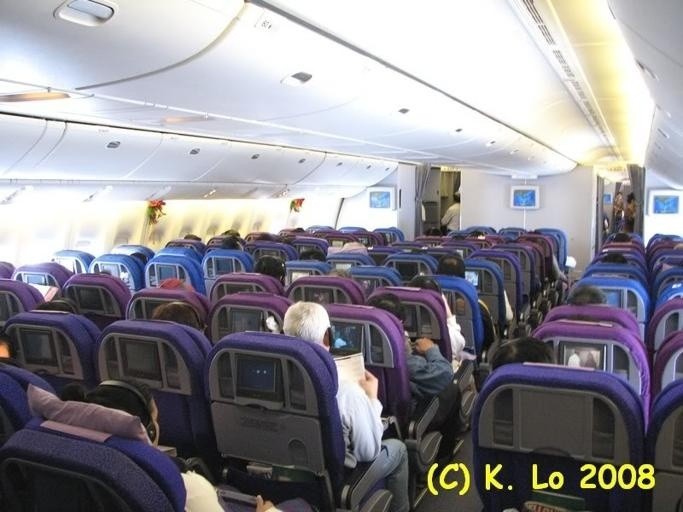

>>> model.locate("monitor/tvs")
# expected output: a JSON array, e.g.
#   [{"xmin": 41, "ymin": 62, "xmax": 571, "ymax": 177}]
[
  {"xmin": 510, "ymin": 185, "xmax": 539, "ymax": 210},
  {"xmin": 366, "ymin": 187, "xmax": 395, "ymax": 212},
  {"xmin": 0, "ymin": 228, "xmax": 623, "ymax": 512},
  {"xmin": 648, "ymin": 190, "xmax": 683, "ymax": 217},
  {"xmin": 602, "ymin": 192, "xmax": 611, "ymax": 204}
]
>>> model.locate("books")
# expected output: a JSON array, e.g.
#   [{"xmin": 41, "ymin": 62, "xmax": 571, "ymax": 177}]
[
  {"xmin": 328, "ymin": 352, "xmax": 368, "ymax": 387},
  {"xmin": 29, "ymin": 282, "xmax": 58, "ymax": 301}
]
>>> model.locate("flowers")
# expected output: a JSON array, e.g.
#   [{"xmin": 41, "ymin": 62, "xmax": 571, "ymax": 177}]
[
  {"xmin": 289, "ymin": 198, "xmax": 304, "ymax": 213},
  {"xmin": 146, "ymin": 200, "xmax": 166, "ymax": 224}
]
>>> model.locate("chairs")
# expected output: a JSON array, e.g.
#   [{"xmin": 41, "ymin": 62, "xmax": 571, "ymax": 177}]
[{"xmin": 0, "ymin": 225, "xmax": 683, "ymax": 512}]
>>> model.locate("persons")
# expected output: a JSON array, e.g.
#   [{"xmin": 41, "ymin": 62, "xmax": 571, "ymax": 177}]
[
  {"xmin": 0, "ymin": 331, "xmax": 19, "ymax": 359},
  {"xmin": 674, "ymin": 239, "xmax": 683, "ymax": 253},
  {"xmin": 159, "ymin": 183, "xmax": 638, "ymax": 475},
  {"xmin": 150, "ymin": 300, "xmax": 208, "ymax": 339},
  {"xmin": 283, "ymin": 300, "xmax": 411, "ymax": 512},
  {"xmin": 60, "ymin": 377, "xmax": 286, "ymax": 512},
  {"xmin": 37, "ymin": 298, "xmax": 81, "ymax": 317}
]
